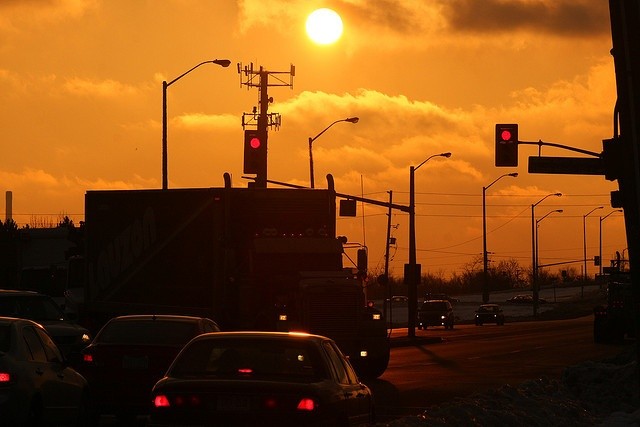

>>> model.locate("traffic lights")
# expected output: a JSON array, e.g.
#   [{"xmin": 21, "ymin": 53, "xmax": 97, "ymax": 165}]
[
  {"xmin": 244, "ymin": 130, "xmax": 268, "ymax": 174},
  {"xmin": 494, "ymin": 123, "xmax": 518, "ymax": 167}
]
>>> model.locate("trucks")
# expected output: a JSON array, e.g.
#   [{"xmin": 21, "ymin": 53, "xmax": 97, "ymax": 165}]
[
  {"xmin": 15, "ymin": 266, "xmax": 86, "ymax": 324},
  {"xmin": 85, "ymin": 188, "xmax": 391, "ymax": 380}
]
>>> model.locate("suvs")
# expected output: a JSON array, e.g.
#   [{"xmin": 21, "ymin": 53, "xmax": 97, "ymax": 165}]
[
  {"xmin": 0, "ymin": 289, "xmax": 92, "ymax": 365},
  {"xmin": 417, "ymin": 300, "xmax": 454, "ymax": 329}
]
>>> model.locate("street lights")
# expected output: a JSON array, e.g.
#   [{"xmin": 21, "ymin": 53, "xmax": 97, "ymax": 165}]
[
  {"xmin": 308, "ymin": 116, "xmax": 359, "ymax": 188},
  {"xmin": 531, "ymin": 192, "xmax": 563, "ymax": 314},
  {"xmin": 482, "ymin": 172, "xmax": 518, "ymax": 302},
  {"xmin": 162, "ymin": 58, "xmax": 231, "ymax": 189},
  {"xmin": 535, "ymin": 209, "xmax": 563, "ymax": 278},
  {"xmin": 600, "ymin": 209, "xmax": 623, "ymax": 274},
  {"xmin": 408, "ymin": 152, "xmax": 452, "ymax": 336},
  {"xmin": 583, "ymin": 206, "xmax": 604, "ymax": 276}
]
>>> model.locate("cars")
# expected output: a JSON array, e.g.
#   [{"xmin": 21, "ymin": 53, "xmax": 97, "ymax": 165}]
[
  {"xmin": 144, "ymin": 331, "xmax": 374, "ymax": 426},
  {"xmin": 474, "ymin": 303, "xmax": 504, "ymax": 327},
  {"xmin": 77, "ymin": 313, "xmax": 223, "ymax": 381},
  {"xmin": 507, "ymin": 294, "xmax": 545, "ymax": 305},
  {"xmin": 424, "ymin": 294, "xmax": 460, "ymax": 303},
  {"xmin": 387, "ymin": 296, "xmax": 408, "ymax": 305},
  {"xmin": 0, "ymin": 316, "xmax": 89, "ymax": 426}
]
